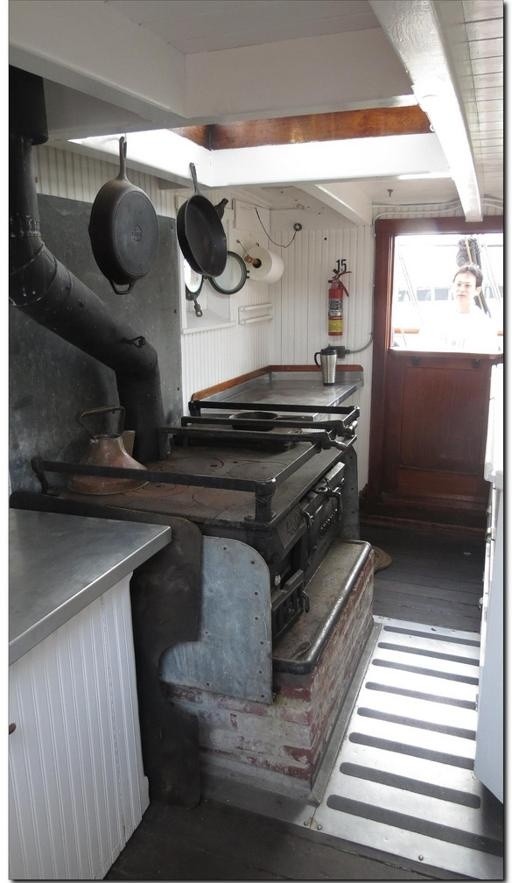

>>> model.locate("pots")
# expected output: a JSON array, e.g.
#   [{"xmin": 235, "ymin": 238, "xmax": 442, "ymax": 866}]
[
  {"xmin": 177, "ymin": 163, "xmax": 250, "ymax": 318},
  {"xmin": 226, "ymin": 411, "xmax": 305, "ymax": 431},
  {"xmin": 88, "ymin": 135, "xmax": 158, "ymax": 295}
]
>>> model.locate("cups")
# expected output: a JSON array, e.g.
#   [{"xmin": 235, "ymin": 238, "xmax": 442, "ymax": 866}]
[{"xmin": 313, "ymin": 348, "xmax": 339, "ymax": 386}]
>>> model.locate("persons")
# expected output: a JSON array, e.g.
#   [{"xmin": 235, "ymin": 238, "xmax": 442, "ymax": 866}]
[{"xmin": 433, "ymin": 263, "xmax": 500, "ymax": 350}]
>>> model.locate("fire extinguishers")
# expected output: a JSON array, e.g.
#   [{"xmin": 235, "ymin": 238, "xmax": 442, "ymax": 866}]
[{"xmin": 327, "ymin": 268, "xmax": 352, "ymax": 335}]
